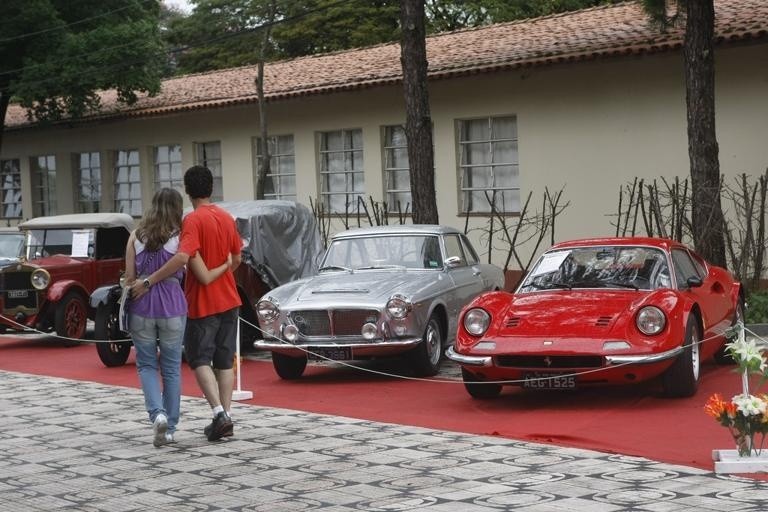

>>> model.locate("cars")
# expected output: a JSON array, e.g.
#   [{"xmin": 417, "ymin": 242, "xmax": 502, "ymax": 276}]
[
  {"xmin": 255, "ymin": 224, "xmax": 505, "ymax": 377},
  {"xmin": 91, "ymin": 200, "xmax": 318, "ymax": 368},
  {"xmin": 0, "ymin": 212, "xmax": 134, "ymax": 346},
  {"xmin": 445, "ymin": 237, "xmax": 743, "ymax": 396}
]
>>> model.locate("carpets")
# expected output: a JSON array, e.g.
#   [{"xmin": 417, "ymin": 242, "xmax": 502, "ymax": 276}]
[{"xmin": 1, "ymin": 336, "xmax": 766, "ymax": 483}]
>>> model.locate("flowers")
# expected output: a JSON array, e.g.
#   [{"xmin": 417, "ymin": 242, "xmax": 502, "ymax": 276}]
[{"xmin": 703, "ymin": 335, "xmax": 767, "ymax": 458}]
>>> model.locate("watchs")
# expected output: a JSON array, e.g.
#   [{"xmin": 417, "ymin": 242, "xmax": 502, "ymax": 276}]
[{"xmin": 143, "ymin": 278, "xmax": 151, "ymax": 289}]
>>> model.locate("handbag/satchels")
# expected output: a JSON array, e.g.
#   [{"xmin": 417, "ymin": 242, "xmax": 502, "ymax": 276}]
[{"xmin": 117, "ymin": 284, "xmax": 130, "ymax": 333}]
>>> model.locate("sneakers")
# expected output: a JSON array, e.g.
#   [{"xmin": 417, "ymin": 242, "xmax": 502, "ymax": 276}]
[
  {"xmin": 165, "ymin": 430, "xmax": 175, "ymax": 442},
  {"xmin": 151, "ymin": 413, "xmax": 168, "ymax": 446}
]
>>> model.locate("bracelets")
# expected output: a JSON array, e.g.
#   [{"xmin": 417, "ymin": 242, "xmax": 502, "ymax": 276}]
[{"xmin": 225, "ymin": 260, "xmax": 230, "ymax": 269}]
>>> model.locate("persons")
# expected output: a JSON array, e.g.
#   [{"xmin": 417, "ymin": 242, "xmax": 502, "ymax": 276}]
[
  {"xmin": 121, "ymin": 187, "xmax": 232, "ymax": 447},
  {"xmin": 125, "ymin": 165, "xmax": 244, "ymax": 441}
]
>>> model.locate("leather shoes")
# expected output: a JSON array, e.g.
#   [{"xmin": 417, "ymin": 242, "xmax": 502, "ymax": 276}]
[
  {"xmin": 205, "ymin": 421, "xmax": 236, "ymax": 435},
  {"xmin": 205, "ymin": 412, "xmax": 233, "ymax": 441}
]
